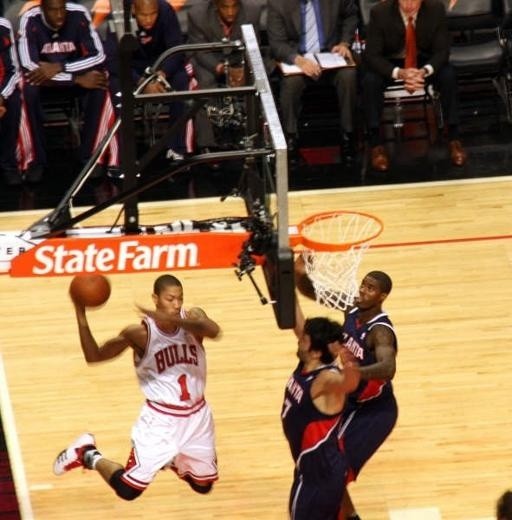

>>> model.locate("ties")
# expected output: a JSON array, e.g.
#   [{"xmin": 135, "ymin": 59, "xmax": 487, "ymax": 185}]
[
  {"xmin": 304, "ymin": 2, "xmax": 321, "ymax": 52},
  {"xmin": 404, "ymin": 17, "xmax": 418, "ymax": 95}
]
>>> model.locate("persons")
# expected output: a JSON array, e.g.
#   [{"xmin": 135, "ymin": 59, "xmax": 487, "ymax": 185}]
[
  {"xmin": 16, "ymin": 0, "xmax": 109, "ymax": 169},
  {"xmin": 297, "ymin": 271, "xmax": 397, "ymax": 520},
  {"xmin": 0, "ymin": 17, "xmax": 22, "ymax": 169},
  {"xmin": 358, "ymin": 1, "xmax": 469, "ymax": 172},
  {"xmin": 281, "ymin": 253, "xmax": 362, "ymax": 519},
  {"xmin": 187, "ymin": 0, "xmax": 260, "ymax": 168},
  {"xmin": 266, "ymin": 0, "xmax": 361, "ymax": 176},
  {"xmin": 52, "ymin": 276, "xmax": 220, "ymax": 501},
  {"xmin": 105, "ymin": 0, "xmax": 189, "ymax": 169}
]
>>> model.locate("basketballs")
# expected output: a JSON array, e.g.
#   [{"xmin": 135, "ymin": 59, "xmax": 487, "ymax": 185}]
[{"xmin": 70, "ymin": 275, "xmax": 110, "ymax": 305}]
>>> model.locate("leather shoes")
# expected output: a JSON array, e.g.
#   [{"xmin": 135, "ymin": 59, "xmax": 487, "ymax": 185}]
[
  {"xmin": 370, "ymin": 144, "xmax": 389, "ymax": 171},
  {"xmin": 337, "ymin": 134, "xmax": 359, "ymax": 166},
  {"xmin": 284, "ymin": 137, "xmax": 301, "ymax": 175},
  {"xmin": 447, "ymin": 140, "xmax": 468, "ymax": 166}
]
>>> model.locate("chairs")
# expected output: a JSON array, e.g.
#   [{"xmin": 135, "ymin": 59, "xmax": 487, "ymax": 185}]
[{"xmin": 1, "ymin": 0, "xmax": 512, "ymax": 189}]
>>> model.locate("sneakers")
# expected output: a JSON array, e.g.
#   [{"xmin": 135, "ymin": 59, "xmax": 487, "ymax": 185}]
[{"xmin": 52, "ymin": 433, "xmax": 97, "ymax": 475}]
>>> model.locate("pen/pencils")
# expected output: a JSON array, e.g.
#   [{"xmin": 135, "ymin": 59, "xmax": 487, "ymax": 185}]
[{"xmin": 313, "ymin": 53, "xmax": 320, "ymax": 65}]
